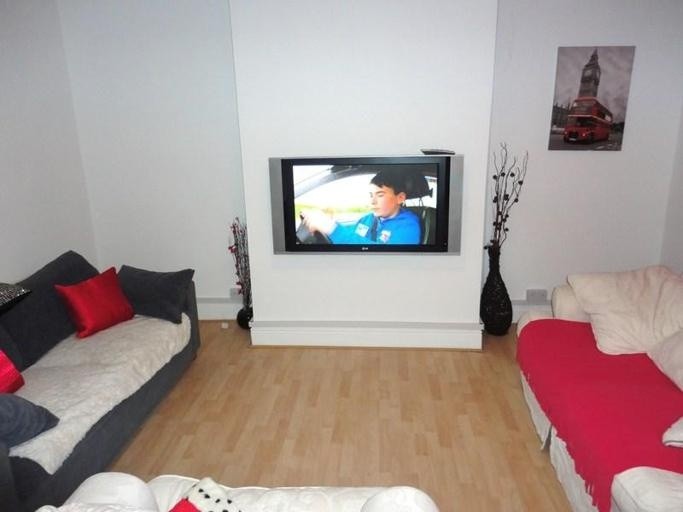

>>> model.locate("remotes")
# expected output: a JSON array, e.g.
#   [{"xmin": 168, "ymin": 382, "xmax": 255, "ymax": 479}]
[{"xmin": 422, "ymin": 149, "xmax": 455, "ymax": 155}]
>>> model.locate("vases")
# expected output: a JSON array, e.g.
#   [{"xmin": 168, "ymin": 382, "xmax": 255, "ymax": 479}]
[
  {"xmin": 237, "ymin": 308, "xmax": 252, "ymax": 329},
  {"xmin": 476, "ymin": 246, "xmax": 512, "ymax": 335}
]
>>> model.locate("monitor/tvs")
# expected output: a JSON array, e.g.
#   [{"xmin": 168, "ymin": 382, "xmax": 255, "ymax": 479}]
[{"xmin": 269, "ymin": 155, "xmax": 463, "ymax": 253}]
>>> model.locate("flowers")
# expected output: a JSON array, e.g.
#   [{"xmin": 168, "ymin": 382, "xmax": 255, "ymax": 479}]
[
  {"xmin": 228, "ymin": 217, "xmax": 252, "ymax": 308},
  {"xmin": 490, "ymin": 144, "xmax": 529, "ymax": 245}
]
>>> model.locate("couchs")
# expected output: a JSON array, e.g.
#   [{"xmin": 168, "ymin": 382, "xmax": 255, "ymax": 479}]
[
  {"xmin": 516, "ymin": 266, "xmax": 683, "ymax": 511},
  {"xmin": 0, "ymin": 252, "xmax": 200, "ymax": 512}
]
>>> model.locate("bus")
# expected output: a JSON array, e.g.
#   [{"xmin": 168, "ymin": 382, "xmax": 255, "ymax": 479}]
[{"xmin": 563, "ymin": 97, "xmax": 613, "ymax": 147}]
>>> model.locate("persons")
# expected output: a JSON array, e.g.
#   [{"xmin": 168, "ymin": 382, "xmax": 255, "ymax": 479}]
[{"xmin": 301, "ymin": 170, "xmax": 422, "ymax": 245}]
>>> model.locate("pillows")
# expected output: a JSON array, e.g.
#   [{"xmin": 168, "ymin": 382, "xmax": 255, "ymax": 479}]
[
  {"xmin": 53, "ymin": 266, "xmax": 136, "ymax": 339},
  {"xmin": 116, "ymin": 260, "xmax": 193, "ymax": 327},
  {"xmin": 0, "ymin": 352, "xmax": 60, "ymax": 453},
  {"xmin": 565, "ymin": 267, "xmax": 682, "ymax": 355}
]
